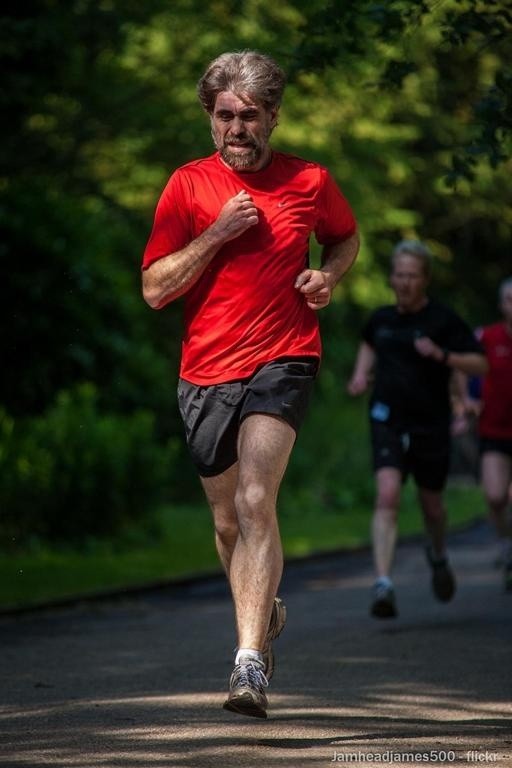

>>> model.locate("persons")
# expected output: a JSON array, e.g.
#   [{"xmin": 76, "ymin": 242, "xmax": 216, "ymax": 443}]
[
  {"xmin": 140, "ymin": 52, "xmax": 364, "ymax": 720},
  {"xmin": 346, "ymin": 240, "xmax": 491, "ymax": 618},
  {"xmin": 456, "ymin": 278, "xmax": 512, "ymax": 593}
]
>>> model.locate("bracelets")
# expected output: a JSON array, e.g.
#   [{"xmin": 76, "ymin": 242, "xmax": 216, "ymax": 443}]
[{"xmin": 442, "ymin": 350, "xmax": 449, "ymax": 364}]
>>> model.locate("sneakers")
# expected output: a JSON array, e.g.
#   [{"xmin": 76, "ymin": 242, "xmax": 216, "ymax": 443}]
[
  {"xmin": 261, "ymin": 597, "xmax": 287, "ymax": 686},
  {"xmin": 223, "ymin": 655, "xmax": 269, "ymax": 718},
  {"xmin": 368, "ymin": 582, "xmax": 398, "ymax": 619},
  {"xmin": 427, "ymin": 547, "xmax": 459, "ymax": 612}
]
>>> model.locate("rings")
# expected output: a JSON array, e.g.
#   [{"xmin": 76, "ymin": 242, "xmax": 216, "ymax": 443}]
[{"xmin": 314, "ymin": 296, "xmax": 318, "ymax": 303}]
